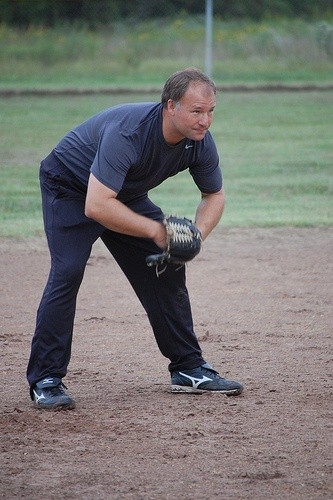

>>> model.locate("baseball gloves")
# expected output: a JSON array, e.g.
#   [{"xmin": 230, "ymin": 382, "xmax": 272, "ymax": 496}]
[{"xmin": 144, "ymin": 213, "xmax": 202, "ymax": 277}]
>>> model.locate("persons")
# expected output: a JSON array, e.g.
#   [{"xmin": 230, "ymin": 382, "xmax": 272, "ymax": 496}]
[{"xmin": 26, "ymin": 67, "xmax": 244, "ymax": 413}]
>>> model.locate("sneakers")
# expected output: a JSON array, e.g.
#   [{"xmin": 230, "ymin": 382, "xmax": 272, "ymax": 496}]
[
  {"xmin": 171, "ymin": 363, "xmax": 244, "ymax": 394},
  {"xmin": 33, "ymin": 377, "xmax": 76, "ymax": 410}
]
